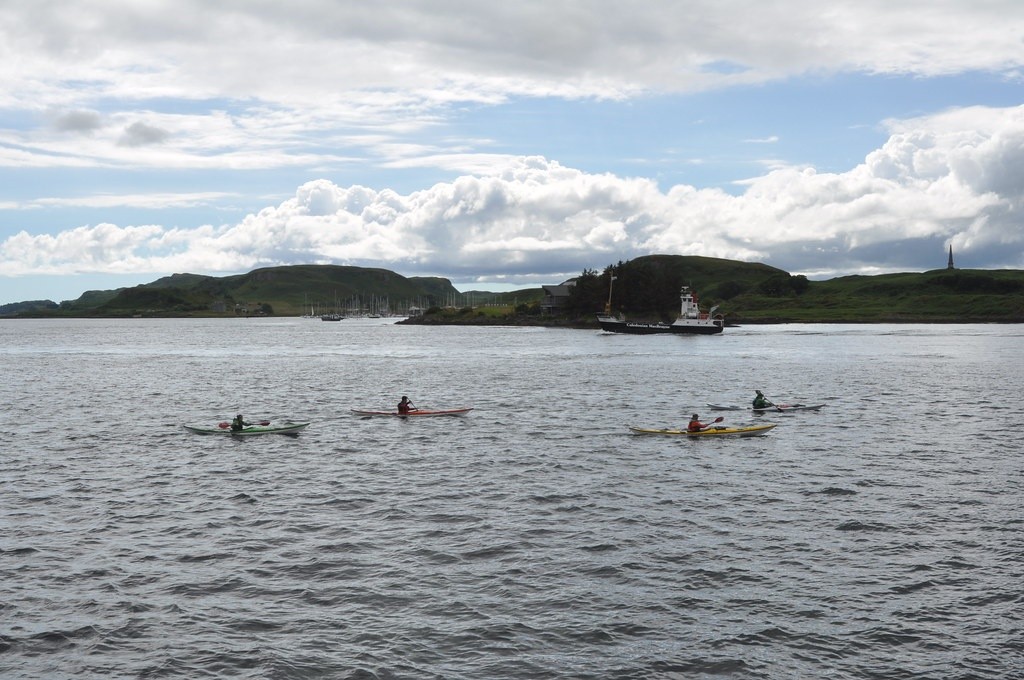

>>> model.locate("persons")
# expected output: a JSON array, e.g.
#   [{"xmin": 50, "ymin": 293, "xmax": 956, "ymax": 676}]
[
  {"xmin": 398, "ymin": 396, "xmax": 416, "ymax": 413},
  {"xmin": 688, "ymin": 414, "xmax": 708, "ymax": 432},
  {"xmin": 231, "ymin": 414, "xmax": 251, "ymax": 431},
  {"xmin": 753, "ymin": 393, "xmax": 774, "ymax": 409}
]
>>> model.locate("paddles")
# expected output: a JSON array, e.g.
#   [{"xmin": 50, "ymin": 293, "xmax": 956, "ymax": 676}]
[
  {"xmin": 406, "ymin": 396, "xmax": 420, "ymax": 412},
  {"xmin": 682, "ymin": 416, "xmax": 725, "ymax": 432},
  {"xmin": 755, "ymin": 388, "xmax": 785, "ymax": 413},
  {"xmin": 219, "ymin": 419, "xmax": 272, "ymax": 428}
]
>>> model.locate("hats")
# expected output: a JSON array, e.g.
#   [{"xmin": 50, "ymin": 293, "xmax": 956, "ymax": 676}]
[
  {"xmin": 237, "ymin": 414, "xmax": 245, "ymax": 419},
  {"xmin": 693, "ymin": 414, "xmax": 700, "ymax": 417},
  {"xmin": 758, "ymin": 393, "xmax": 765, "ymax": 397}
]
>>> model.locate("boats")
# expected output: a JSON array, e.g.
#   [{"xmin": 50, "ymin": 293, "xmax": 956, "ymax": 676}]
[
  {"xmin": 629, "ymin": 423, "xmax": 778, "ymax": 436},
  {"xmin": 182, "ymin": 423, "xmax": 311, "ymax": 435},
  {"xmin": 705, "ymin": 402, "xmax": 826, "ymax": 412},
  {"xmin": 597, "ymin": 270, "xmax": 724, "ymax": 335},
  {"xmin": 350, "ymin": 407, "xmax": 474, "ymax": 416}
]
